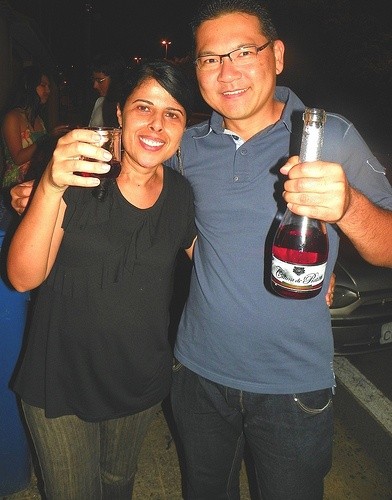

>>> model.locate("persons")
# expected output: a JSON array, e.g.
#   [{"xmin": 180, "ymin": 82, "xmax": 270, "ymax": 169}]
[
  {"xmin": 84, "ymin": 59, "xmax": 129, "ymax": 131},
  {"xmin": 6, "ymin": 58, "xmax": 337, "ymax": 500},
  {"xmin": 10, "ymin": 1, "xmax": 392, "ymax": 499},
  {"xmin": 1, "ymin": 69, "xmax": 71, "ymax": 225}
]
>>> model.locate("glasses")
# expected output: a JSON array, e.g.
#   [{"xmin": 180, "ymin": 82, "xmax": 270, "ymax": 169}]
[
  {"xmin": 91, "ymin": 78, "xmax": 105, "ymax": 83},
  {"xmin": 193, "ymin": 41, "xmax": 280, "ymax": 70}
]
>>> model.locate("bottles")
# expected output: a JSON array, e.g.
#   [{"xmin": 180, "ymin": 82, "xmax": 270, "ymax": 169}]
[{"xmin": 269, "ymin": 109, "xmax": 329, "ymax": 299}]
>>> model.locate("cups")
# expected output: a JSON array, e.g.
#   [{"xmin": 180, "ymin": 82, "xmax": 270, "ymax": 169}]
[{"xmin": 78, "ymin": 127, "xmax": 122, "ymax": 179}]
